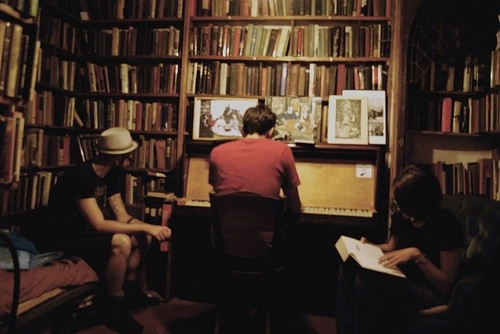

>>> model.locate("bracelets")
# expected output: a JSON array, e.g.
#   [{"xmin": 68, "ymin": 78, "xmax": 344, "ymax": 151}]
[{"xmin": 415, "ymin": 260, "xmax": 428, "ymax": 264}]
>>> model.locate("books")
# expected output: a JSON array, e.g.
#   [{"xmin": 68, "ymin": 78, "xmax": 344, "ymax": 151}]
[
  {"xmin": 410, "ymin": 50, "xmax": 500, "ymax": 93},
  {"xmin": 187, "ymin": 61, "xmax": 389, "ymax": 97},
  {"xmin": 432, "ymin": 158, "xmax": 500, "ymax": 202},
  {"xmin": 0, "ymin": 0, "xmax": 185, "ymax": 102},
  {"xmin": 334, "ymin": 235, "xmax": 406, "ymax": 278},
  {"xmin": 0, "ymin": 91, "xmax": 177, "ymax": 217},
  {"xmin": 189, "ymin": 23, "xmax": 392, "ymax": 58},
  {"xmin": 189, "ymin": 0, "xmax": 395, "ymax": 17},
  {"xmin": 408, "ymin": 93, "xmax": 500, "ymax": 134}
]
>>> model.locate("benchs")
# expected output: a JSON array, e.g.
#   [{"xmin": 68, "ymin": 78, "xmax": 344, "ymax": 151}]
[{"xmin": 0, "ymin": 203, "xmax": 145, "ymax": 334}]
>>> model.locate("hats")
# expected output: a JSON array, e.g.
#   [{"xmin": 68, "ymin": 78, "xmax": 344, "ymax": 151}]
[{"xmin": 94, "ymin": 126, "xmax": 138, "ymax": 155}]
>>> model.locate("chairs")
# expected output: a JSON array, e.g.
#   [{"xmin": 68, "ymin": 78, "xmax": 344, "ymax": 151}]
[{"xmin": 210, "ymin": 192, "xmax": 284, "ymax": 334}]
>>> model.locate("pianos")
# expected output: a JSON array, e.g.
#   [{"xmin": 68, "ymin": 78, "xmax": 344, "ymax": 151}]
[{"xmin": 163, "ymin": 137, "xmax": 384, "ymax": 241}]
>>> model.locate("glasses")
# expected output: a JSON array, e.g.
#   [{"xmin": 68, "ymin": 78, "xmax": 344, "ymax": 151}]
[{"xmin": 390, "ymin": 200, "xmax": 399, "ymax": 214}]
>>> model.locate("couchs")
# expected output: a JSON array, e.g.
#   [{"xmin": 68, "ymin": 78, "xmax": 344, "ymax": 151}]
[{"xmin": 336, "ymin": 195, "xmax": 500, "ymax": 334}]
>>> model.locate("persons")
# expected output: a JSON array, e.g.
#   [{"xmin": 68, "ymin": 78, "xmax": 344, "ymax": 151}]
[
  {"xmin": 335, "ymin": 164, "xmax": 465, "ymax": 334},
  {"xmin": 46, "ymin": 127, "xmax": 172, "ymax": 300},
  {"xmin": 208, "ymin": 104, "xmax": 302, "ymax": 319}
]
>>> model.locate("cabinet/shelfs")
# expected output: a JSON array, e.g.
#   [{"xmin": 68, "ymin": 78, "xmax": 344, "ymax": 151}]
[
  {"xmin": 405, "ymin": 49, "xmax": 500, "ymax": 202},
  {"xmin": 0, "ymin": 0, "xmax": 402, "ymax": 292}
]
